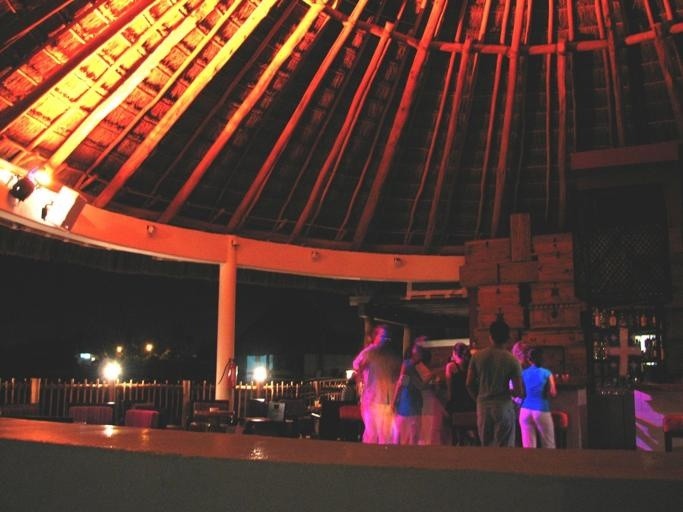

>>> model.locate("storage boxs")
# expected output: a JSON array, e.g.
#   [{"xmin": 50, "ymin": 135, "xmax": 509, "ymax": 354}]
[{"xmin": 457, "ymin": 212, "xmax": 587, "ymax": 387}]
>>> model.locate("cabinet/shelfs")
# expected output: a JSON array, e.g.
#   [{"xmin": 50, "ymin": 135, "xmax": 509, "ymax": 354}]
[
  {"xmin": 590, "ymin": 301, "xmax": 665, "ymax": 368},
  {"xmin": 552, "ymin": 411, "xmax": 569, "ymax": 449}
]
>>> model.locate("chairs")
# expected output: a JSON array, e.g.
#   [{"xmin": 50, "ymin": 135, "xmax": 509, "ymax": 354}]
[{"xmin": 69, "ymin": 400, "xmax": 360, "ymax": 434}]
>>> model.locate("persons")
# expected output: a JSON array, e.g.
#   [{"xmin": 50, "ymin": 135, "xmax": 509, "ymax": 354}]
[
  {"xmin": 389, "ymin": 335, "xmax": 558, "ymax": 449},
  {"xmin": 464, "ymin": 320, "xmax": 526, "ymax": 448},
  {"xmin": 352, "ymin": 323, "xmax": 402, "ymax": 445}
]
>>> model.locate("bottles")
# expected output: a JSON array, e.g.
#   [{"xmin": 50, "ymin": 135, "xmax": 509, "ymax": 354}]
[{"xmin": 588, "ymin": 306, "xmax": 663, "ymax": 396}]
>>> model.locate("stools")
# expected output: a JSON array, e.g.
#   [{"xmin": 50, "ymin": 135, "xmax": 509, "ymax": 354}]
[
  {"xmin": 662, "ymin": 414, "xmax": 683, "ymax": 451},
  {"xmin": 451, "ymin": 411, "xmax": 476, "ymax": 445}
]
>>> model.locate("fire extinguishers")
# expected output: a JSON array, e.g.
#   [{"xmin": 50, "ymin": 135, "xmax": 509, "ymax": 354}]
[{"xmin": 219, "ymin": 358, "xmax": 238, "ymax": 388}]
